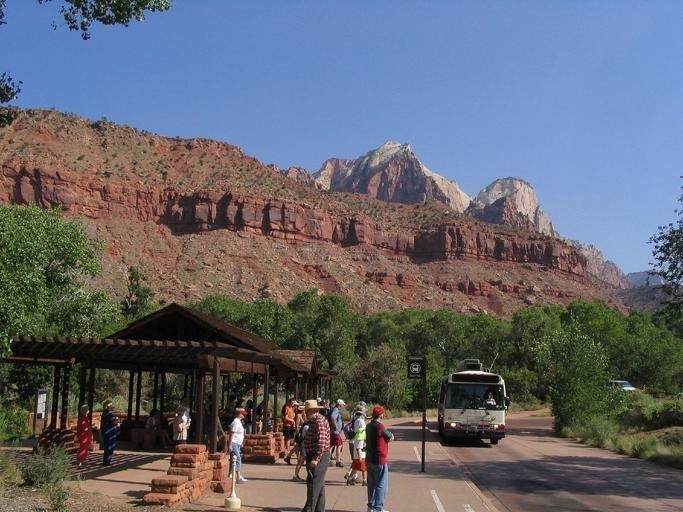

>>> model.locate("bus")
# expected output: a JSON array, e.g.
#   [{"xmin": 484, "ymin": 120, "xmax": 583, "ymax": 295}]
[{"xmin": 436, "ymin": 357, "xmax": 512, "ymax": 446}]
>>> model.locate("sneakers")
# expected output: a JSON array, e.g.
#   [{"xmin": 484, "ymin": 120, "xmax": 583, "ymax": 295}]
[
  {"xmin": 374, "ymin": 507, "xmax": 391, "ymax": 512},
  {"xmin": 336, "ymin": 462, "xmax": 345, "ymax": 467},
  {"xmin": 332, "ymin": 455, "xmax": 337, "ymax": 460},
  {"xmin": 293, "ymin": 476, "xmax": 306, "ymax": 482},
  {"xmin": 228, "ymin": 473, "xmax": 238, "ymax": 478},
  {"xmin": 235, "ymin": 477, "xmax": 248, "ymax": 484},
  {"xmin": 284, "ymin": 457, "xmax": 292, "ymax": 466},
  {"xmin": 344, "ymin": 472, "xmax": 360, "ymax": 486},
  {"xmin": 362, "ymin": 481, "xmax": 368, "ymax": 487},
  {"xmin": 367, "ymin": 507, "xmax": 374, "ymax": 512}
]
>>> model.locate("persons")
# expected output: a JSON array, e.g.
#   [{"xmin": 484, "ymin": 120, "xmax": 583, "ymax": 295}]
[
  {"xmin": 77, "ymin": 403, "xmax": 91, "ymax": 465},
  {"xmin": 99, "ymin": 400, "xmax": 118, "ymax": 465},
  {"xmin": 460, "ymin": 390, "xmax": 496, "ymax": 409},
  {"xmin": 145, "ymin": 388, "xmax": 370, "ymax": 485},
  {"xmin": 297, "ymin": 399, "xmax": 330, "ymax": 512},
  {"xmin": 365, "ymin": 405, "xmax": 394, "ymax": 512}
]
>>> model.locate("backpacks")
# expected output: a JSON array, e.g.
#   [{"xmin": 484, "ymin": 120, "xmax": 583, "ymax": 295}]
[{"xmin": 343, "ymin": 416, "xmax": 362, "ymax": 440}]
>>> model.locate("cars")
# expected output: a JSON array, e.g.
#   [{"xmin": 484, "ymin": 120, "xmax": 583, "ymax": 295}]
[{"xmin": 608, "ymin": 379, "xmax": 636, "ymax": 392}]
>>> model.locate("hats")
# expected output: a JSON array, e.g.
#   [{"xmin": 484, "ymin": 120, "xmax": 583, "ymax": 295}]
[
  {"xmin": 297, "ymin": 400, "xmax": 328, "ymax": 411},
  {"xmin": 371, "ymin": 406, "xmax": 385, "ymax": 419},
  {"xmin": 337, "ymin": 398, "xmax": 368, "ymax": 414},
  {"xmin": 235, "ymin": 408, "xmax": 248, "ymax": 415}
]
